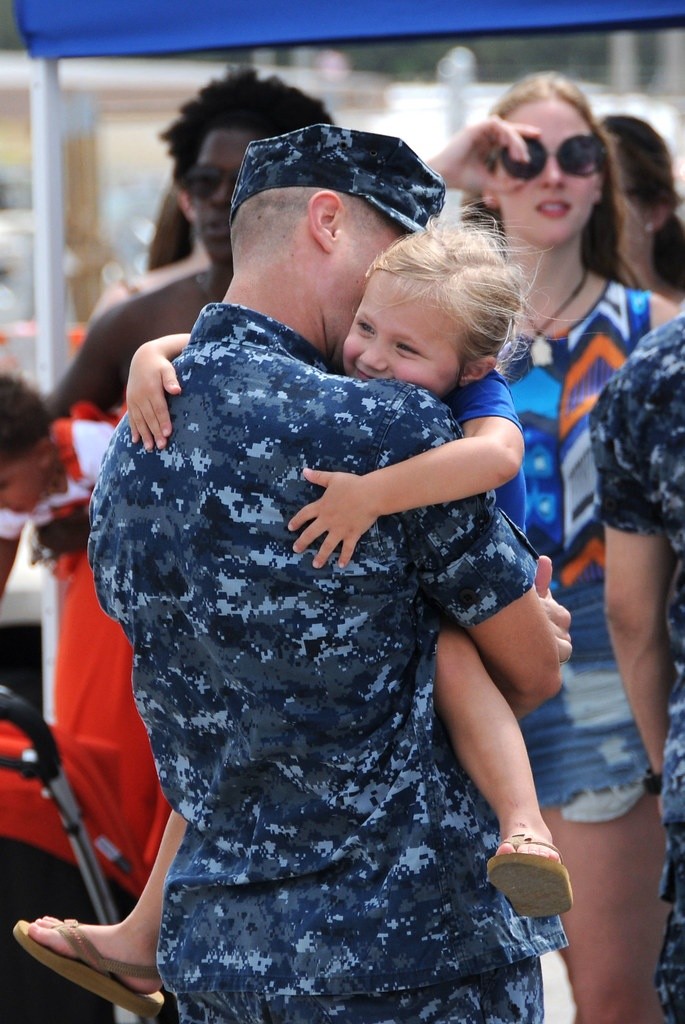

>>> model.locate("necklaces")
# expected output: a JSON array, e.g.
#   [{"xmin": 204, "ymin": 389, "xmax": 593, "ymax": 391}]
[{"xmin": 517, "ymin": 266, "xmax": 589, "ymax": 367}]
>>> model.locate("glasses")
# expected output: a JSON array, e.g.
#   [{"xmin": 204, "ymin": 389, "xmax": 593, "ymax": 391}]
[
  {"xmin": 498, "ymin": 134, "xmax": 606, "ymax": 181},
  {"xmin": 179, "ymin": 164, "xmax": 240, "ymax": 200}
]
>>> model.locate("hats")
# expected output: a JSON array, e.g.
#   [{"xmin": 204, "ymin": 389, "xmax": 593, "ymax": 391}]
[{"xmin": 228, "ymin": 124, "xmax": 445, "ymax": 231}]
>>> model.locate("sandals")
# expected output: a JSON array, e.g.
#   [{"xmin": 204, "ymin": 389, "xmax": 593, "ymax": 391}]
[
  {"xmin": 13, "ymin": 919, "xmax": 163, "ymax": 1018},
  {"xmin": 487, "ymin": 834, "xmax": 572, "ymax": 918}
]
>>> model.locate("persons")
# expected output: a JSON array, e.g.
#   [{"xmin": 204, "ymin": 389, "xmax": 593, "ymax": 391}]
[
  {"xmin": 86, "ymin": 124, "xmax": 576, "ymax": 1024},
  {"xmin": 85, "ymin": 178, "xmax": 210, "ymax": 338},
  {"xmin": 578, "ymin": 98, "xmax": 684, "ymax": 320},
  {"xmin": 15, "ymin": 216, "xmax": 578, "ymax": 1013},
  {"xmin": 590, "ymin": 288, "xmax": 684, "ymax": 1024},
  {"xmin": 33, "ymin": 72, "xmax": 344, "ymax": 415},
  {"xmin": 403, "ymin": 70, "xmax": 680, "ymax": 1023},
  {"xmin": 0, "ymin": 377, "xmax": 124, "ymax": 613}
]
